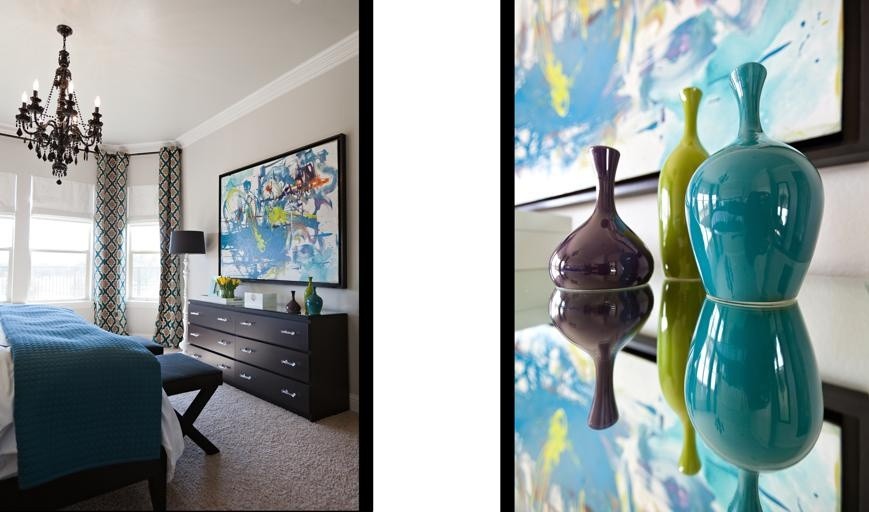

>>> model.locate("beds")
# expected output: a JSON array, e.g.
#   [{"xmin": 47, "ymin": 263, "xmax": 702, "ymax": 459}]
[{"xmin": 0, "ymin": 301, "xmax": 171, "ymax": 512}]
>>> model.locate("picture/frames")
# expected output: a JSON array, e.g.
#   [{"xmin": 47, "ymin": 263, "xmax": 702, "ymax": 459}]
[
  {"xmin": 514, "ymin": 0, "xmax": 869, "ymax": 208},
  {"xmin": 548, "ymin": 317, "xmax": 868, "ymax": 512},
  {"xmin": 217, "ymin": 133, "xmax": 347, "ymax": 289}
]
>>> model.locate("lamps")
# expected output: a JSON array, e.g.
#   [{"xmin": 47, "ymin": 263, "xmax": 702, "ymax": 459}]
[
  {"xmin": 169, "ymin": 230, "xmax": 206, "ymax": 359},
  {"xmin": 13, "ymin": 24, "xmax": 103, "ymax": 185}
]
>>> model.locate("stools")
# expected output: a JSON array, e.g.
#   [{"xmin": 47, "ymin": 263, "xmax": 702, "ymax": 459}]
[
  {"xmin": 155, "ymin": 353, "xmax": 223, "ymax": 456},
  {"xmin": 118, "ymin": 335, "xmax": 164, "ymax": 355}
]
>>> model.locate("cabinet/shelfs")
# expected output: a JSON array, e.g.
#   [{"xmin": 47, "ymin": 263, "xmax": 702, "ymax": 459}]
[
  {"xmin": 186, "ymin": 298, "xmax": 235, "ymax": 387},
  {"xmin": 235, "ymin": 304, "xmax": 350, "ymax": 423}
]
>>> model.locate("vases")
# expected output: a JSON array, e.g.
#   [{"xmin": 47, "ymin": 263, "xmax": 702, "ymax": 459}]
[
  {"xmin": 658, "ymin": 282, "xmax": 707, "ymax": 477},
  {"xmin": 547, "ymin": 285, "xmax": 654, "ymax": 431},
  {"xmin": 304, "ymin": 276, "xmax": 315, "ymax": 315},
  {"xmin": 690, "ymin": 62, "xmax": 824, "ymax": 307},
  {"xmin": 683, "ymin": 301, "xmax": 825, "ymax": 512},
  {"xmin": 549, "ymin": 146, "xmax": 654, "ymax": 293},
  {"xmin": 657, "ymin": 85, "xmax": 712, "ymax": 281},
  {"xmin": 305, "ymin": 286, "xmax": 323, "ymax": 316},
  {"xmin": 285, "ymin": 290, "xmax": 301, "ymax": 313}
]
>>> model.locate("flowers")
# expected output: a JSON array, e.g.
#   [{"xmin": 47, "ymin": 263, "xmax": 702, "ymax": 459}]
[{"xmin": 218, "ymin": 276, "xmax": 241, "ymax": 289}]
